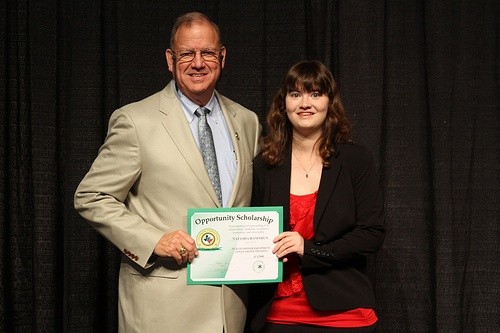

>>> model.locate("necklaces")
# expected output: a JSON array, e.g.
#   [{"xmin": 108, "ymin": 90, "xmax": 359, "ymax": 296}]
[{"xmin": 290, "ymin": 145, "xmax": 321, "ymax": 178}]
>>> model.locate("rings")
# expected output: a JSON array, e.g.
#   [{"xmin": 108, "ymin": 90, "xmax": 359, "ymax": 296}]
[{"xmin": 179, "ymin": 248, "xmax": 187, "ymax": 256}]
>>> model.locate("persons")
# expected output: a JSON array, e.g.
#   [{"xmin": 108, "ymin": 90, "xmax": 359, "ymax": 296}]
[
  {"xmin": 72, "ymin": 11, "xmax": 265, "ymax": 333},
  {"xmin": 252, "ymin": 58, "xmax": 384, "ymax": 333}
]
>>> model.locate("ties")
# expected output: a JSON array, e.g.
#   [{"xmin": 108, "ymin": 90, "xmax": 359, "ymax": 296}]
[{"xmin": 194, "ymin": 107, "xmax": 222, "ymax": 208}]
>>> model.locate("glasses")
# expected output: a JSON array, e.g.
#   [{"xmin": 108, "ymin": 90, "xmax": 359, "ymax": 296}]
[{"xmin": 172, "ymin": 49, "xmax": 222, "ymax": 62}]
